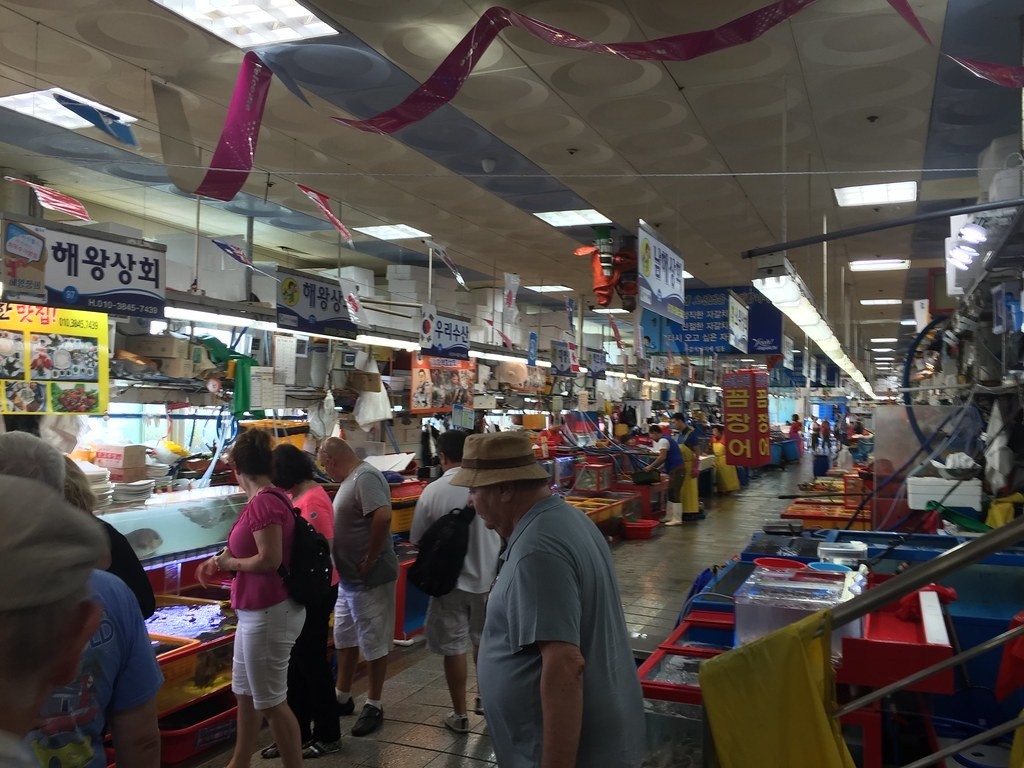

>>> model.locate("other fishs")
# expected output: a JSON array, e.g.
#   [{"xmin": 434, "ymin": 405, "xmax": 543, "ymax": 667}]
[{"xmin": 122, "ymin": 492, "xmax": 249, "ymax": 559}]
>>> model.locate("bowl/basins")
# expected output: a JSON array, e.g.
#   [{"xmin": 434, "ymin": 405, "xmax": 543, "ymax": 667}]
[
  {"xmin": 936, "ymin": 466, "xmax": 984, "ymax": 481},
  {"xmin": 752, "ymin": 557, "xmax": 808, "ymax": 571},
  {"xmin": 52, "ymin": 351, "xmax": 72, "ymax": 370},
  {"xmin": 808, "ymin": 562, "xmax": 854, "ymax": 571},
  {"xmin": 622, "ymin": 519, "xmax": 660, "ymax": 539}
]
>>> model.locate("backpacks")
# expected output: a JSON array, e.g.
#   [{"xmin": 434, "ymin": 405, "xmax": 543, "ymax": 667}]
[{"xmin": 260, "ymin": 489, "xmax": 333, "ymax": 604}]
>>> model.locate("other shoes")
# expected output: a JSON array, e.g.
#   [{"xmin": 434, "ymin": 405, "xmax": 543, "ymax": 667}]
[
  {"xmin": 444, "ymin": 710, "xmax": 470, "ymax": 731},
  {"xmin": 351, "ymin": 704, "xmax": 383, "ymax": 736},
  {"xmin": 336, "ymin": 696, "xmax": 355, "ymax": 714},
  {"xmin": 471, "ymin": 696, "xmax": 483, "ymax": 712}
]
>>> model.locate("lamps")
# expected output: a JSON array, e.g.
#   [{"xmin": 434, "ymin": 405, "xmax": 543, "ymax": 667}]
[{"xmin": 750, "ymin": 76, "xmax": 1018, "ymax": 402}]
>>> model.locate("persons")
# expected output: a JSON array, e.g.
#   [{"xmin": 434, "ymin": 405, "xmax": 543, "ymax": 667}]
[
  {"xmin": 789, "ymin": 413, "xmax": 863, "ymax": 471},
  {"xmin": 260, "ymin": 444, "xmax": 341, "ymax": 758},
  {"xmin": 671, "ymin": 413, "xmax": 700, "ymax": 517},
  {"xmin": 0, "ymin": 430, "xmax": 166, "ymax": 768},
  {"xmin": 704, "ymin": 425, "xmax": 740, "ymax": 494},
  {"xmin": 448, "ymin": 431, "xmax": 646, "ymax": 768},
  {"xmin": 196, "ymin": 427, "xmax": 307, "ymax": 768},
  {"xmin": 643, "ymin": 425, "xmax": 686, "ymax": 526},
  {"xmin": 410, "ymin": 429, "xmax": 501, "ymax": 732},
  {"xmin": 318, "ymin": 436, "xmax": 398, "ymax": 736}
]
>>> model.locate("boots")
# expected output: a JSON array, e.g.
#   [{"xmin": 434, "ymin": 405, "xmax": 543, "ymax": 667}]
[
  {"xmin": 665, "ymin": 503, "xmax": 683, "ymax": 525},
  {"xmin": 658, "ymin": 501, "xmax": 674, "ymax": 521}
]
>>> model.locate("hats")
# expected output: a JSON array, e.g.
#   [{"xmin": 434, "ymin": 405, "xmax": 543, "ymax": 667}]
[
  {"xmin": 448, "ymin": 433, "xmax": 551, "ymax": 489},
  {"xmin": 1, "ymin": 475, "xmax": 112, "ymax": 609}
]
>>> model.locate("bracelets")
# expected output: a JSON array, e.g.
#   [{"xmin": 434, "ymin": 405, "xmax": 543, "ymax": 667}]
[{"xmin": 366, "ymin": 556, "xmax": 378, "ymax": 564}]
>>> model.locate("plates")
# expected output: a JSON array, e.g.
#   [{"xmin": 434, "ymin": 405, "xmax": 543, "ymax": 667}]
[{"xmin": 6, "ymin": 382, "xmax": 44, "ymax": 412}]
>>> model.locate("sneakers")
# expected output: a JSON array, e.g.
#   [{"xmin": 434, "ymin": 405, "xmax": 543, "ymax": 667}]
[
  {"xmin": 261, "ymin": 738, "xmax": 313, "ymax": 757},
  {"xmin": 301, "ymin": 736, "xmax": 343, "ymax": 757}
]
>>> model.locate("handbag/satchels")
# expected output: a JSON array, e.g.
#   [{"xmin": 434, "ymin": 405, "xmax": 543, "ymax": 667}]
[{"xmin": 406, "ymin": 506, "xmax": 470, "ymax": 597}]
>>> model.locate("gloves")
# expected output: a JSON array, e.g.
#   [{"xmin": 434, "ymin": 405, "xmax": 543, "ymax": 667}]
[{"xmin": 691, "ymin": 458, "xmax": 701, "ymax": 478}]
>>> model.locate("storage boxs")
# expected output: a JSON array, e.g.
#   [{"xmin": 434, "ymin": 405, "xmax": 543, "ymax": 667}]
[{"xmin": 0, "ymin": 212, "xmax": 1024, "ymax": 768}]
